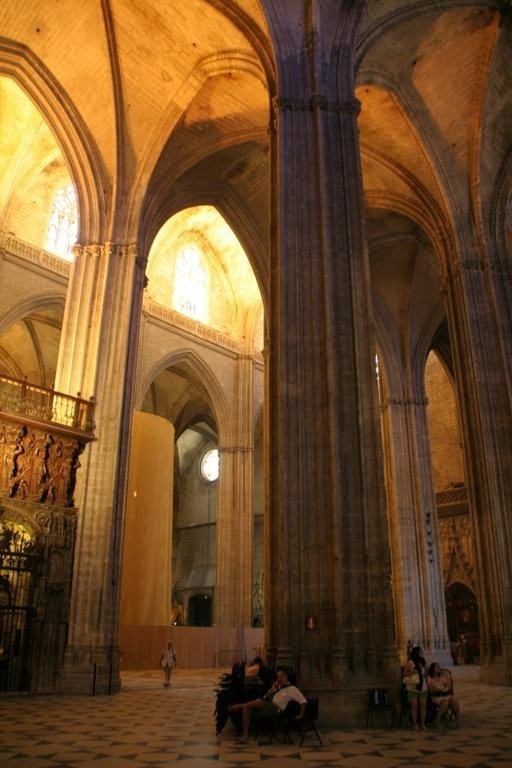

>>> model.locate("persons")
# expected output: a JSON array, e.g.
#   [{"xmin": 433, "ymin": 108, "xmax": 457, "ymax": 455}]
[
  {"xmin": 159, "ymin": 641, "xmax": 178, "ymax": 687},
  {"xmin": 426, "ymin": 662, "xmax": 463, "ymax": 727},
  {"xmin": 457, "ymin": 636, "xmax": 474, "ymax": 664},
  {"xmin": 228, "ymin": 667, "xmax": 308, "ymax": 744},
  {"xmin": 403, "ymin": 646, "xmax": 428, "ymax": 730}
]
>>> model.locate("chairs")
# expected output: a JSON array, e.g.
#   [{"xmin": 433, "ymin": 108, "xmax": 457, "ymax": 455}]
[
  {"xmin": 251, "ymin": 699, "xmax": 322, "ymax": 746},
  {"xmin": 399, "ymin": 669, "xmax": 460, "ymax": 729},
  {"xmin": 367, "ymin": 688, "xmax": 397, "ymax": 729}
]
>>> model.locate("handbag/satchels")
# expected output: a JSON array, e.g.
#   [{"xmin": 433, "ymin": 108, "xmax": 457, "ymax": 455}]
[
  {"xmin": 403, "ymin": 673, "xmax": 420, "ymax": 685},
  {"xmin": 432, "ymin": 689, "xmax": 453, "ymax": 697}
]
